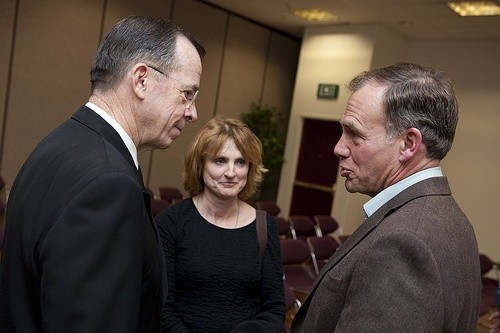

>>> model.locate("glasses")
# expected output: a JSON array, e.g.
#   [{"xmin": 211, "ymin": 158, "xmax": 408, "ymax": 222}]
[{"xmin": 147, "ymin": 65, "xmax": 199, "ymax": 110}]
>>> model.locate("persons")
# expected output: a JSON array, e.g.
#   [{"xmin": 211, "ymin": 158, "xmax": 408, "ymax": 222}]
[
  {"xmin": 153, "ymin": 113, "xmax": 287, "ymax": 333},
  {"xmin": 290, "ymin": 61, "xmax": 483, "ymax": 333},
  {"xmin": 0, "ymin": 15, "xmax": 207, "ymax": 333}
]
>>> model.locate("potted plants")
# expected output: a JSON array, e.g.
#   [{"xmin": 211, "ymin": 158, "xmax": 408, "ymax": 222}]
[{"xmin": 240, "ymin": 97, "xmax": 286, "ymax": 201}]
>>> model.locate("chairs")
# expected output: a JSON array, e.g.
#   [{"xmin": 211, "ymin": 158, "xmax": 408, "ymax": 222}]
[{"xmin": 143, "ymin": 186, "xmax": 500, "ymax": 333}]
